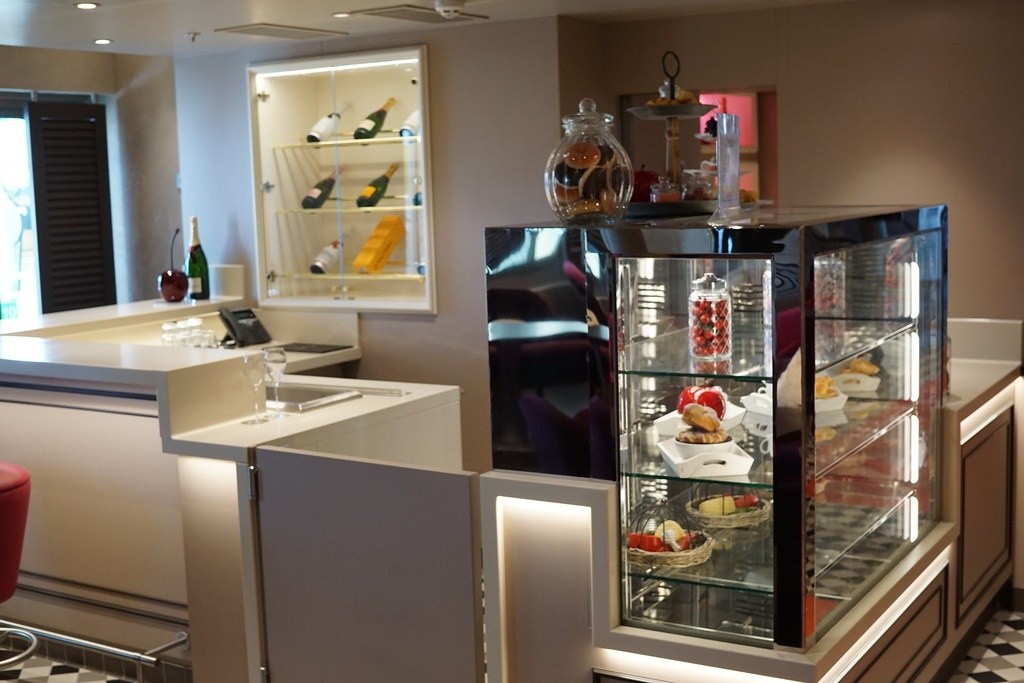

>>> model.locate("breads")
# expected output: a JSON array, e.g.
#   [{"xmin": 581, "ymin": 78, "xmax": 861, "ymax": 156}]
[
  {"xmin": 815, "ymin": 375, "xmax": 839, "ymax": 399},
  {"xmin": 840, "ymin": 358, "xmax": 882, "ymax": 385}
]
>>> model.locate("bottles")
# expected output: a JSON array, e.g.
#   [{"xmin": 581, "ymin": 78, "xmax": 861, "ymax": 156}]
[
  {"xmin": 185, "ymin": 216, "xmax": 209, "ymax": 299},
  {"xmin": 650, "ymin": 176, "xmax": 681, "ymax": 202},
  {"xmin": 307, "ymin": 104, "xmax": 346, "ymax": 148},
  {"xmin": 311, "ymin": 225, "xmax": 352, "ymax": 274},
  {"xmin": 354, "ymin": 97, "xmax": 395, "ymax": 146},
  {"xmin": 357, "ymin": 162, "xmax": 400, "ymax": 213},
  {"xmin": 681, "ymin": 172, "xmax": 714, "ymax": 200},
  {"xmin": 302, "ymin": 166, "xmax": 344, "ymax": 214},
  {"xmin": 687, "ymin": 273, "xmax": 732, "ymax": 360},
  {"xmin": 544, "ymin": 97, "xmax": 634, "ymax": 226},
  {"xmin": 706, "ymin": 112, "xmax": 750, "ymax": 225}
]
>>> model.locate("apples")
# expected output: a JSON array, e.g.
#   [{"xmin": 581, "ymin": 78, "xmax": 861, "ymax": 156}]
[{"xmin": 157, "ymin": 228, "xmax": 189, "ymax": 303}]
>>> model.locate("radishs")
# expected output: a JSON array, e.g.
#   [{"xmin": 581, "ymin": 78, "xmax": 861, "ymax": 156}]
[
  {"xmin": 699, "ymin": 496, "xmax": 736, "ymax": 516},
  {"xmin": 654, "ymin": 520, "xmax": 685, "ymax": 542}
]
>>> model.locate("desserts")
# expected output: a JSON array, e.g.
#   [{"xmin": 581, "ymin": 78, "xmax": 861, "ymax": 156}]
[{"xmin": 673, "ymin": 385, "xmax": 734, "ymax": 459}]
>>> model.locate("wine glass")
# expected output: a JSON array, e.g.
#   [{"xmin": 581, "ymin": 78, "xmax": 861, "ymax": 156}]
[
  {"xmin": 241, "ymin": 351, "xmax": 269, "ymax": 424},
  {"xmin": 264, "ymin": 347, "xmax": 291, "ymax": 417}
]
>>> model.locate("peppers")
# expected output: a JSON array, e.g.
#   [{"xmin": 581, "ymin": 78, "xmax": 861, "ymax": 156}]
[
  {"xmin": 733, "ymin": 490, "xmax": 760, "ymax": 507},
  {"xmin": 629, "ymin": 531, "xmax": 701, "ymax": 553}
]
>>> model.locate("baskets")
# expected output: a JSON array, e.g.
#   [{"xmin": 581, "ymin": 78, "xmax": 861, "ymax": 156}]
[
  {"xmin": 687, "ymin": 493, "xmax": 770, "ymax": 528},
  {"xmin": 621, "ymin": 530, "xmax": 715, "ymax": 569}
]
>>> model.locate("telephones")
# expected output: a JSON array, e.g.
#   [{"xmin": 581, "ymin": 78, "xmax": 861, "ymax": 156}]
[{"xmin": 219, "ymin": 306, "xmax": 272, "ymax": 348}]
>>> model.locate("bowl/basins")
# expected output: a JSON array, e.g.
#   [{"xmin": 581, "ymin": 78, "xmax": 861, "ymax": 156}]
[{"xmin": 674, "ymin": 435, "xmax": 734, "ymax": 459}]
